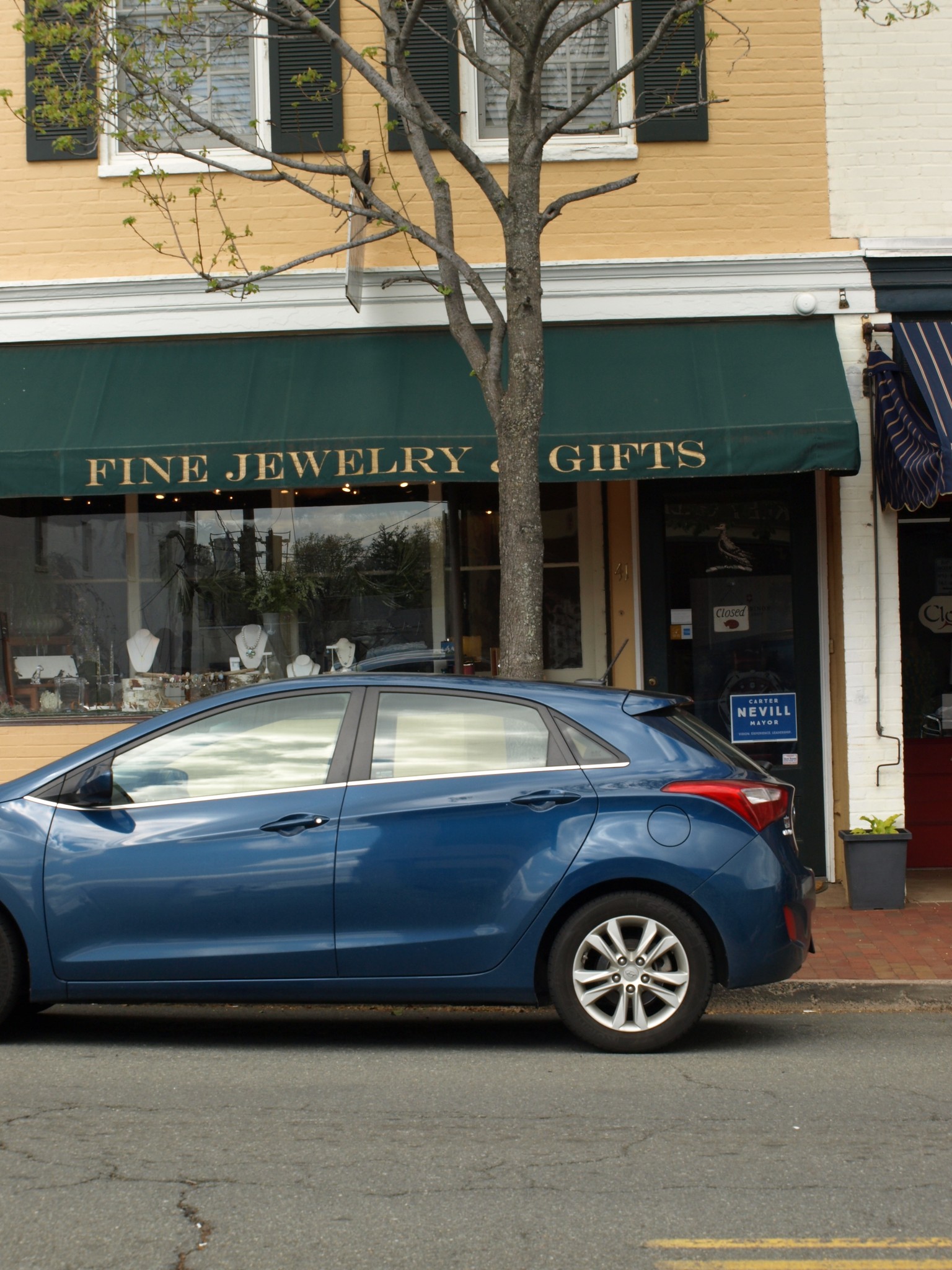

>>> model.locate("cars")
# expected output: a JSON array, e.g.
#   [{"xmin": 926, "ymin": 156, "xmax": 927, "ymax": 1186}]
[
  {"xmin": 0, "ymin": 639, "xmax": 815, "ymax": 1054},
  {"xmin": 331, "ymin": 642, "xmax": 492, "ymax": 677}
]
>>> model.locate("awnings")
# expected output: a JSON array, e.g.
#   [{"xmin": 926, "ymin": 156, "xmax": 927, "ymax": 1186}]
[
  {"xmin": 871, "ymin": 317, "xmax": 951, "ymax": 513},
  {"xmin": 1, "ymin": 312, "xmax": 863, "ymax": 504}
]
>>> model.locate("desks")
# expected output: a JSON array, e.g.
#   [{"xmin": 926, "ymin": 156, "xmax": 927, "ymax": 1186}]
[{"xmin": 13, "ymin": 683, "xmax": 88, "ymax": 713}]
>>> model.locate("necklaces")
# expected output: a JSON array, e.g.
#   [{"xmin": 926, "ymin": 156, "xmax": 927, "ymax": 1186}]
[
  {"xmin": 291, "ymin": 661, "xmax": 315, "ymax": 677},
  {"xmin": 241, "ymin": 625, "xmax": 261, "ymax": 659},
  {"xmin": 335, "ymin": 642, "xmax": 351, "ymax": 665},
  {"xmin": 133, "ymin": 634, "xmax": 154, "ymax": 661}
]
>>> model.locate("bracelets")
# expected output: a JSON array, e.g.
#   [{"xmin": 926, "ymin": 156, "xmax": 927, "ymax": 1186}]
[{"xmin": 155, "ymin": 669, "xmax": 224, "ymax": 691}]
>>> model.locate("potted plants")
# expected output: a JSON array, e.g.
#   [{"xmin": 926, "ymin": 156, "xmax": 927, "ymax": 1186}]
[{"xmin": 838, "ymin": 813, "xmax": 913, "ymax": 911}]
